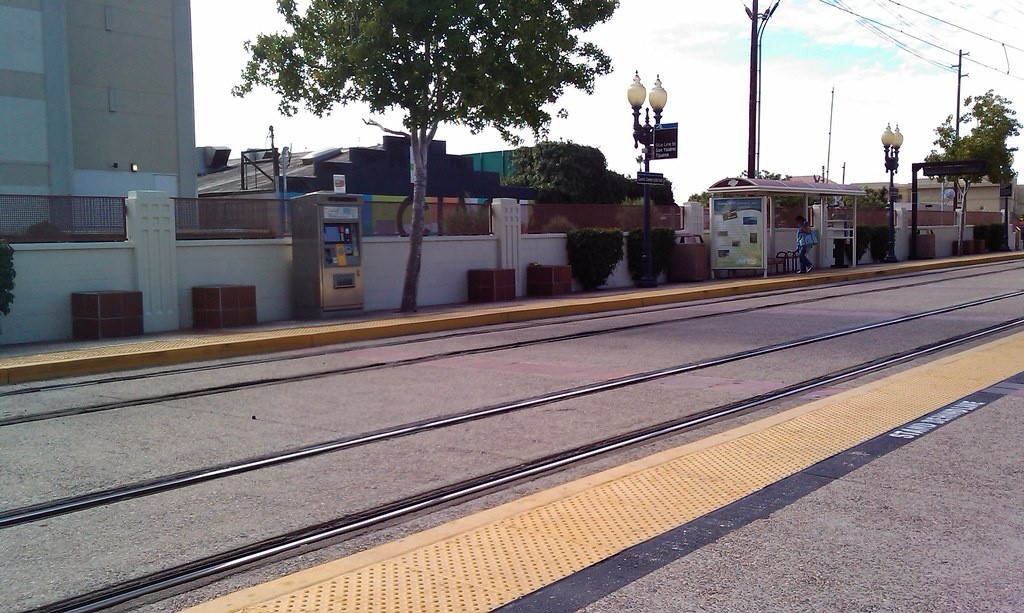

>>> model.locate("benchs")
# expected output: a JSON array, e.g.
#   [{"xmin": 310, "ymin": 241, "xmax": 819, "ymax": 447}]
[{"xmin": 769, "ymin": 249, "xmax": 800, "ymax": 274}]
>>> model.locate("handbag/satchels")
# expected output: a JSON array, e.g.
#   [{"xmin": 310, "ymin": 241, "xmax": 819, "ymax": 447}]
[{"xmin": 800, "ymin": 222, "xmax": 817, "ymax": 246}]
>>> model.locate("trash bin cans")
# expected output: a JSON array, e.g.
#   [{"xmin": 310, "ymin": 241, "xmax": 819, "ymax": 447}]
[
  {"xmin": 909, "ymin": 228, "xmax": 936, "ymax": 259},
  {"xmin": 665, "ymin": 233, "xmax": 708, "ymax": 282}
]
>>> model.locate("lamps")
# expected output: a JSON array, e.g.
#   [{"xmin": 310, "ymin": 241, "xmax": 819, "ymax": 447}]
[{"xmin": 132, "ymin": 163, "xmax": 138, "ymax": 172}]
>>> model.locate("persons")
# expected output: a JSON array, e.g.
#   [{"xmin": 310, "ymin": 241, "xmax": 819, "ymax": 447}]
[{"xmin": 795, "ymin": 216, "xmax": 814, "ymax": 274}]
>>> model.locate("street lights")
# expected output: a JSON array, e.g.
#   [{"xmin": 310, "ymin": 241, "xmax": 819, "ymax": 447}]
[
  {"xmin": 626, "ymin": 69, "xmax": 668, "ymax": 289},
  {"xmin": 880, "ymin": 121, "xmax": 903, "ymax": 264}
]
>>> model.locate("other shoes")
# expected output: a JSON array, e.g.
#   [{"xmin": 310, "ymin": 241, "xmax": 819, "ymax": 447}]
[
  {"xmin": 807, "ymin": 265, "xmax": 814, "ymax": 272},
  {"xmin": 797, "ymin": 269, "xmax": 805, "ymax": 273}
]
